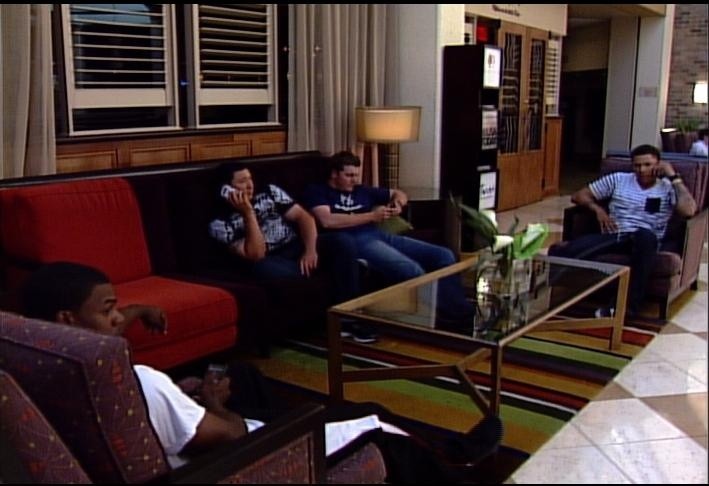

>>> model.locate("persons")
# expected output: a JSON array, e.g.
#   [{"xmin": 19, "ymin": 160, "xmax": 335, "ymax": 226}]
[
  {"xmin": 26, "ymin": 260, "xmax": 504, "ymax": 485},
  {"xmin": 688, "ymin": 128, "xmax": 708, "ymax": 156},
  {"xmin": 559, "ymin": 144, "xmax": 699, "ymax": 319},
  {"xmin": 208, "ymin": 161, "xmax": 380, "ymax": 344},
  {"xmin": 309, "ymin": 150, "xmax": 464, "ymax": 311}
]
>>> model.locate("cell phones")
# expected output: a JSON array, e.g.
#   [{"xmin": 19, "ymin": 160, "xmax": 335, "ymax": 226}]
[
  {"xmin": 205, "ymin": 360, "xmax": 229, "ymax": 385},
  {"xmin": 221, "ymin": 184, "xmax": 240, "ymax": 201}
]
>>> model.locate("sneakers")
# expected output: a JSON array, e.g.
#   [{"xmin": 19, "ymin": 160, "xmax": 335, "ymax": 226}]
[
  {"xmin": 339, "ymin": 322, "xmax": 380, "ymax": 343},
  {"xmin": 592, "ymin": 299, "xmax": 618, "ymax": 319},
  {"xmin": 433, "ymin": 410, "xmax": 506, "ymax": 472}
]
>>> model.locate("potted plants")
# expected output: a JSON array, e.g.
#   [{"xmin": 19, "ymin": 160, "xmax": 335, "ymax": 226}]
[{"xmin": 456, "ymin": 203, "xmax": 549, "ymax": 297}]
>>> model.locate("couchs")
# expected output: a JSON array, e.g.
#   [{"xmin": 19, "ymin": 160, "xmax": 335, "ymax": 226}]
[{"xmin": 1, "ymin": 151, "xmax": 462, "ymax": 371}]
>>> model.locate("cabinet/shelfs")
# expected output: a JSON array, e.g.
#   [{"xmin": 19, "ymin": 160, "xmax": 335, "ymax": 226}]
[
  {"xmin": 547, "ymin": 33, "xmax": 560, "ymax": 108},
  {"xmin": 466, "ymin": 14, "xmax": 497, "ymax": 45},
  {"xmin": 544, "ymin": 115, "xmax": 562, "ymax": 196},
  {"xmin": 497, "ymin": 21, "xmax": 545, "ymax": 212},
  {"xmin": 444, "ymin": 46, "xmax": 497, "ymax": 203}
]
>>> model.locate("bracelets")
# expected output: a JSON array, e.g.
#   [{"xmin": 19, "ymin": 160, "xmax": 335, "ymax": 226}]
[
  {"xmin": 672, "ymin": 178, "xmax": 683, "ymax": 185},
  {"xmin": 669, "ymin": 173, "xmax": 680, "ymax": 181}
]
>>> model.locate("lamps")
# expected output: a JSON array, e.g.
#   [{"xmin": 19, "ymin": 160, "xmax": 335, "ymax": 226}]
[
  {"xmin": 356, "ymin": 106, "xmax": 421, "ymax": 189},
  {"xmin": 693, "ymin": 80, "xmax": 708, "ymax": 130}
]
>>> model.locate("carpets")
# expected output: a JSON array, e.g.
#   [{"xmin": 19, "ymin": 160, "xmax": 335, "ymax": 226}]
[{"xmin": 188, "ymin": 284, "xmax": 700, "ymax": 485}]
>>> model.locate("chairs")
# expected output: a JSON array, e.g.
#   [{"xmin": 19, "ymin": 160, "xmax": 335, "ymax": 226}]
[
  {"xmin": 548, "ymin": 149, "xmax": 709, "ymax": 320},
  {"xmin": 661, "ymin": 129, "xmax": 707, "ymax": 154},
  {"xmin": 1, "ymin": 312, "xmax": 395, "ymax": 484}
]
